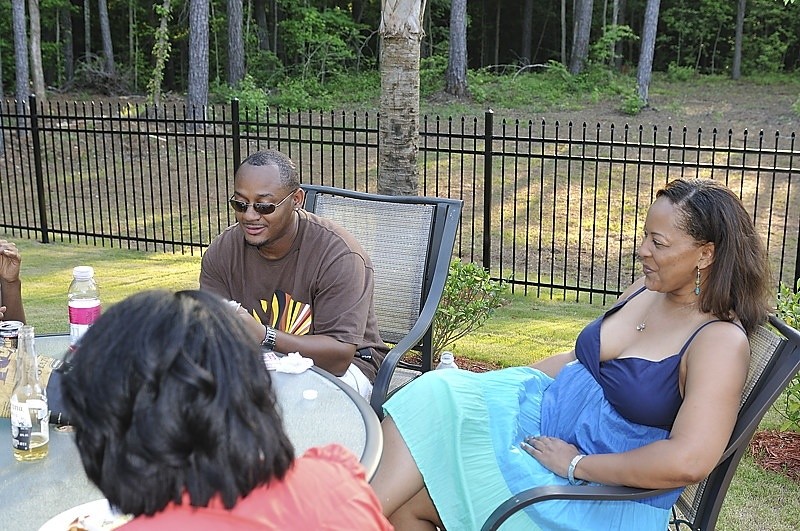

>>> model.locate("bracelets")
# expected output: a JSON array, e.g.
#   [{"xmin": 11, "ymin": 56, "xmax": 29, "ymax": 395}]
[
  {"xmin": 260, "ymin": 324, "xmax": 276, "ymax": 351},
  {"xmin": 568, "ymin": 454, "xmax": 583, "ymax": 486}
]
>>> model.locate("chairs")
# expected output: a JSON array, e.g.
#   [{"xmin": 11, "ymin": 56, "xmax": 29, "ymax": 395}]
[
  {"xmin": 476, "ymin": 309, "xmax": 800, "ymax": 531},
  {"xmin": 303, "ymin": 183, "xmax": 464, "ymax": 414}
]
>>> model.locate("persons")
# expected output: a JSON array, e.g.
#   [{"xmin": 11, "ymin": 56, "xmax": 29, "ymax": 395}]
[
  {"xmin": 57, "ymin": 288, "xmax": 394, "ymax": 531},
  {"xmin": 199, "ymin": 151, "xmax": 391, "ymax": 398},
  {"xmin": 368, "ymin": 178, "xmax": 770, "ymax": 531},
  {"xmin": 0, "ymin": 240, "xmax": 27, "ymax": 326}
]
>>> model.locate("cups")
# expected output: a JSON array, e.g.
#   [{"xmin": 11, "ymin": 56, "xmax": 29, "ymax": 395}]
[{"xmin": 12, "ymin": 325, "xmax": 50, "ymax": 461}]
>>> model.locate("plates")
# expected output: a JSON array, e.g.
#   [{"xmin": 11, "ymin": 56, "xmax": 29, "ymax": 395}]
[{"xmin": 38, "ymin": 499, "xmax": 136, "ymax": 531}]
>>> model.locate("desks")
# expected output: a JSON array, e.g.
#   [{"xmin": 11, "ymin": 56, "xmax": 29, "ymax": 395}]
[{"xmin": 0, "ymin": 333, "xmax": 386, "ymax": 531}]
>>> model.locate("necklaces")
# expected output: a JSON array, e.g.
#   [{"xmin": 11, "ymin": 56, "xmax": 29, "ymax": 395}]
[{"xmin": 636, "ymin": 294, "xmax": 696, "ymax": 331}]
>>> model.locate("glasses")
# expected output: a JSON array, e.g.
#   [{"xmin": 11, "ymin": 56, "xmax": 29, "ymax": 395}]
[{"xmin": 230, "ymin": 190, "xmax": 296, "ymax": 214}]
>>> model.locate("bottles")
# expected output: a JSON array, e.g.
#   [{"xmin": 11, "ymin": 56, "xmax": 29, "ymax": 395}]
[
  {"xmin": 67, "ymin": 265, "xmax": 102, "ymax": 344},
  {"xmin": 435, "ymin": 351, "xmax": 458, "ymax": 370}
]
